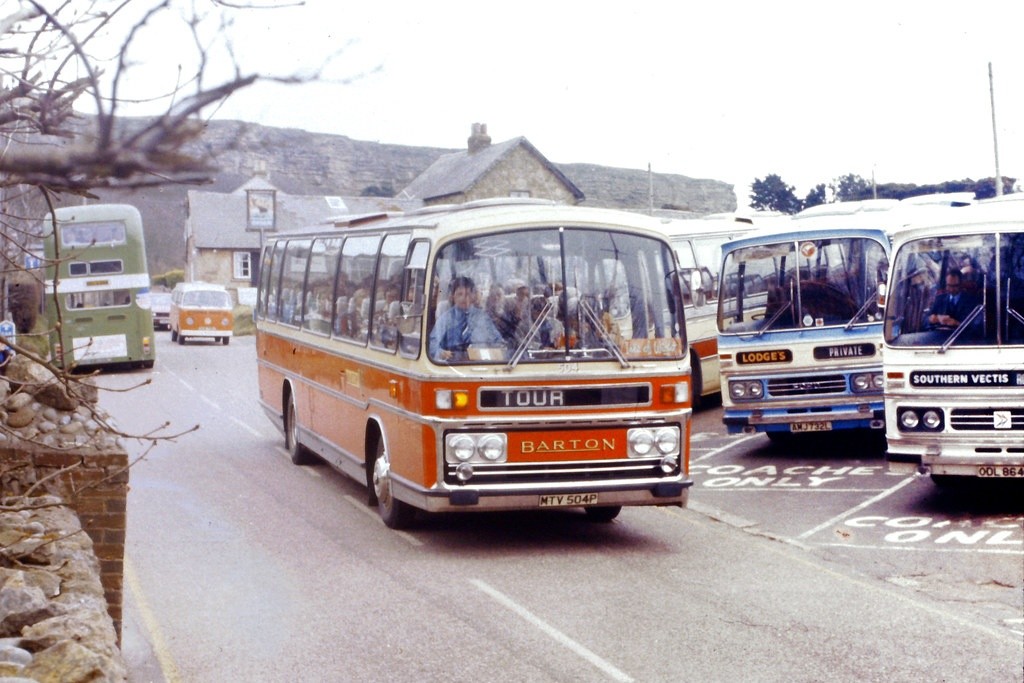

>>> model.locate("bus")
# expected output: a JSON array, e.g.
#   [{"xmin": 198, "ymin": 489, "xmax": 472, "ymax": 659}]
[
  {"xmin": 253, "ymin": 196, "xmax": 712, "ymax": 530},
  {"xmin": 660, "ymin": 190, "xmax": 975, "ymax": 442},
  {"xmin": 876, "ymin": 191, "xmax": 1023, "ymax": 488},
  {"xmin": 44, "ymin": 203, "xmax": 155, "ymax": 369},
  {"xmin": 482, "ymin": 211, "xmax": 794, "ymax": 414}
]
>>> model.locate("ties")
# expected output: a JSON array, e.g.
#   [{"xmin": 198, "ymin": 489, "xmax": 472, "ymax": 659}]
[
  {"xmin": 950, "ymin": 295, "xmax": 955, "ymax": 308},
  {"xmin": 462, "ymin": 311, "xmax": 471, "ymax": 347}
]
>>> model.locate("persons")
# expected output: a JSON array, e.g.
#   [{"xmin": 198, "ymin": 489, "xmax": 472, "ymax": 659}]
[
  {"xmin": 585, "ymin": 311, "xmax": 618, "ymax": 351},
  {"xmin": 764, "ymin": 268, "xmax": 848, "ymax": 321},
  {"xmin": 382, "ymin": 284, "xmax": 404, "ymax": 328},
  {"xmin": 430, "ymin": 278, "xmax": 515, "ymax": 362},
  {"xmin": 512, "ymin": 280, "xmax": 568, "ymax": 355},
  {"xmin": 290, "ymin": 270, "xmax": 372, "ymax": 343},
  {"xmin": 843, "ymin": 260, "xmax": 862, "ymax": 306},
  {"xmin": 916, "ymin": 269, "xmax": 981, "ymax": 346}
]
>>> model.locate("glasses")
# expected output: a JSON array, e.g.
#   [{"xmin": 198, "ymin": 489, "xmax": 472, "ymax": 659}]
[
  {"xmin": 946, "ymin": 284, "xmax": 960, "ymax": 288},
  {"xmin": 532, "ymin": 304, "xmax": 549, "ymax": 311}
]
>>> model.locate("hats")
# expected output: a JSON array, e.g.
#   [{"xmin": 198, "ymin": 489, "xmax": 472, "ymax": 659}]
[{"xmin": 514, "ymin": 280, "xmax": 529, "ymax": 290}]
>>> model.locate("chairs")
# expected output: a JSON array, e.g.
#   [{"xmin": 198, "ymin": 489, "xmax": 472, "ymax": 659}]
[{"xmin": 278, "ymin": 290, "xmax": 386, "ymax": 349}]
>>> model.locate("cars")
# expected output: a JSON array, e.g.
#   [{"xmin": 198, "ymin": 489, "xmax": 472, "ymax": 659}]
[{"xmin": 152, "ymin": 293, "xmax": 172, "ymax": 329}]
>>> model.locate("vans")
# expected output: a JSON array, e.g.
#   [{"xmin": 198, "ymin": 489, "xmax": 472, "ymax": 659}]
[{"xmin": 169, "ymin": 281, "xmax": 233, "ymax": 345}]
